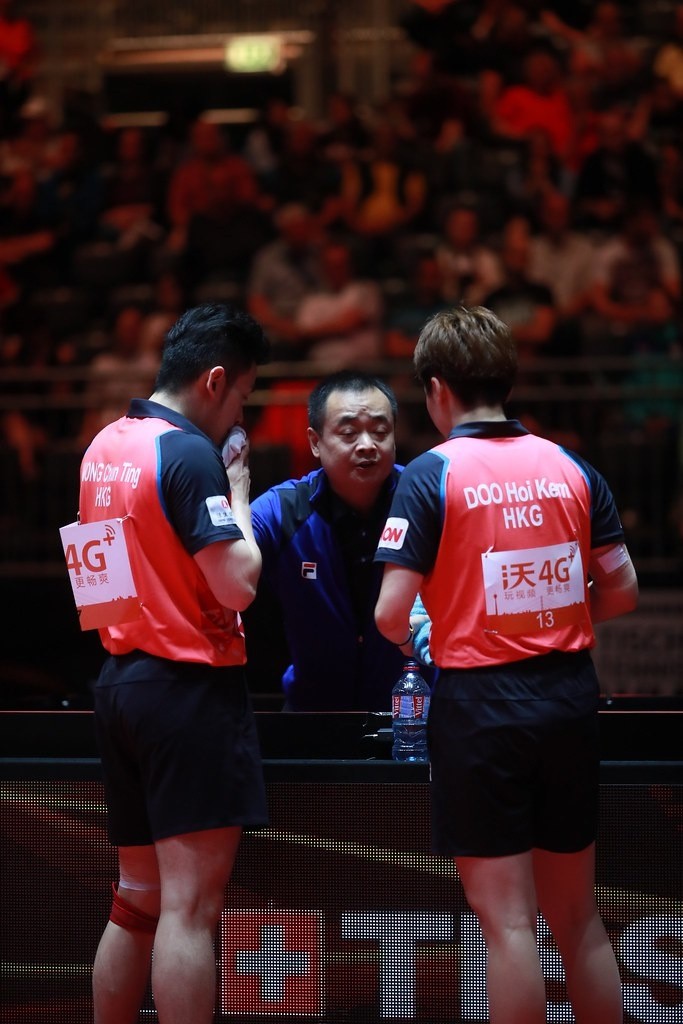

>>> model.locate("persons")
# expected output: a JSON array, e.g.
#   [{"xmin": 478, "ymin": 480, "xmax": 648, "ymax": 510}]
[
  {"xmin": 374, "ymin": 300, "xmax": 638, "ymax": 1024},
  {"xmin": 248, "ymin": 371, "xmax": 441, "ymax": 784},
  {"xmin": 0, "ymin": 0, "xmax": 683, "ymax": 593},
  {"xmin": 59, "ymin": 302, "xmax": 268, "ymax": 1024}
]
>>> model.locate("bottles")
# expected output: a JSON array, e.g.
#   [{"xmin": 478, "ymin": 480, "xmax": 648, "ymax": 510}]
[{"xmin": 391, "ymin": 659, "xmax": 432, "ymax": 766}]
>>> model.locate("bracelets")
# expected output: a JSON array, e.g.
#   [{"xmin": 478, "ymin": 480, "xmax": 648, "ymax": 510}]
[{"xmin": 393, "ymin": 622, "xmax": 414, "ymax": 648}]
